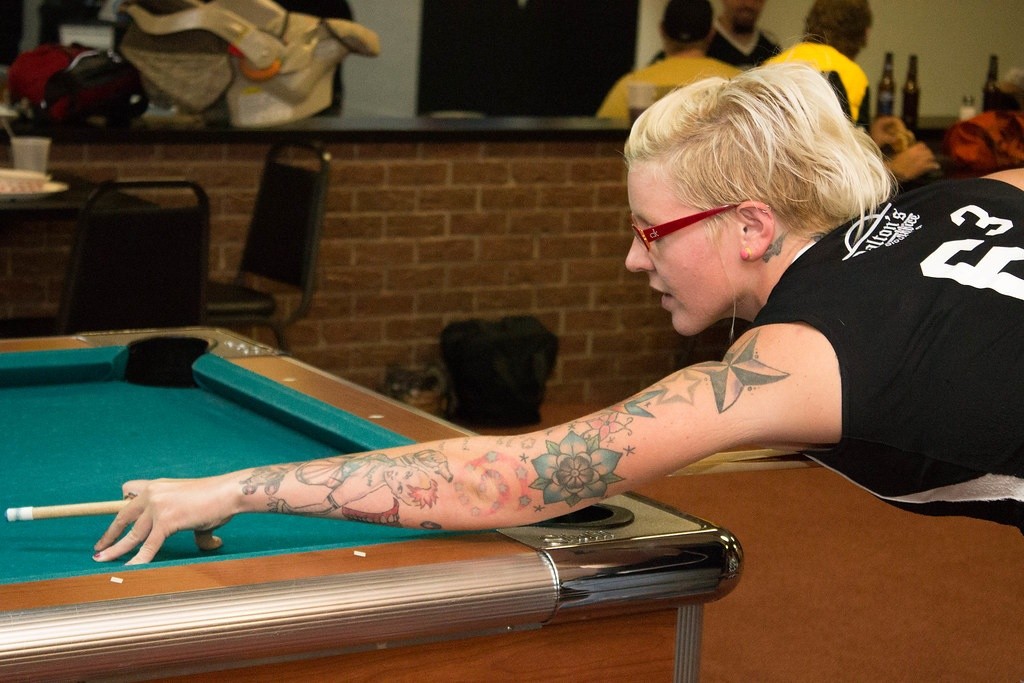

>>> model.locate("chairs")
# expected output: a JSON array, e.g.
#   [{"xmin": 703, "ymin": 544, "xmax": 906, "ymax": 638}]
[
  {"xmin": 0, "ymin": 176, "xmax": 210, "ymax": 338},
  {"xmin": 207, "ymin": 136, "xmax": 333, "ymax": 354}
]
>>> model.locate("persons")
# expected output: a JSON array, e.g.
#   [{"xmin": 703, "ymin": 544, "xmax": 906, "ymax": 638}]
[
  {"xmin": 90, "ymin": 65, "xmax": 1024, "ymax": 568},
  {"xmin": 594, "ymin": 0, "xmax": 1024, "ymax": 188}
]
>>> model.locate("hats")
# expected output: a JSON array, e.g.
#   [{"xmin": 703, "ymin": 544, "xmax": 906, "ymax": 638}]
[{"xmin": 663, "ymin": 0, "xmax": 713, "ymax": 41}]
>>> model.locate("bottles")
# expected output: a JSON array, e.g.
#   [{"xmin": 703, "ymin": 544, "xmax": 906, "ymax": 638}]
[
  {"xmin": 902, "ymin": 53, "xmax": 919, "ymax": 131},
  {"xmin": 959, "ymin": 95, "xmax": 977, "ymax": 121},
  {"xmin": 982, "ymin": 54, "xmax": 998, "ymax": 114},
  {"xmin": 876, "ymin": 51, "xmax": 895, "ymax": 123}
]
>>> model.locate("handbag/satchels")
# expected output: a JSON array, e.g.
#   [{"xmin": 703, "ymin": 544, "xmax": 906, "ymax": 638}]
[
  {"xmin": 440, "ymin": 315, "xmax": 558, "ymax": 424},
  {"xmin": 944, "ymin": 109, "xmax": 1024, "ymax": 173},
  {"xmin": 42, "ymin": 48, "xmax": 149, "ymax": 129}
]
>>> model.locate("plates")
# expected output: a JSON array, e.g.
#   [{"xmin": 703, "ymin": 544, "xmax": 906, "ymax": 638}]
[{"xmin": 0, "ymin": 172, "xmax": 69, "ymax": 205}]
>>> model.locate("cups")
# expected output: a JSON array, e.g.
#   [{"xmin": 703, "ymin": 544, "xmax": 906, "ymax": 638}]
[
  {"xmin": 625, "ymin": 86, "xmax": 655, "ymax": 126},
  {"xmin": 10, "ymin": 138, "xmax": 52, "ymax": 175}
]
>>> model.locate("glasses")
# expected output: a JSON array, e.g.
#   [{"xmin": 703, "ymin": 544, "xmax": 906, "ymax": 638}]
[{"xmin": 630, "ymin": 203, "xmax": 740, "ymax": 251}]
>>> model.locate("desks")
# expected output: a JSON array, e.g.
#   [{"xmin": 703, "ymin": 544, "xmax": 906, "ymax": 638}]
[
  {"xmin": 0, "ymin": 326, "xmax": 745, "ymax": 683},
  {"xmin": 0, "ymin": 172, "xmax": 156, "ymax": 217}
]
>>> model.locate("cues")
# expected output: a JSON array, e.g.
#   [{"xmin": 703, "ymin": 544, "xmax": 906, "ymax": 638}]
[{"xmin": 3, "ymin": 444, "xmax": 828, "ymax": 525}]
